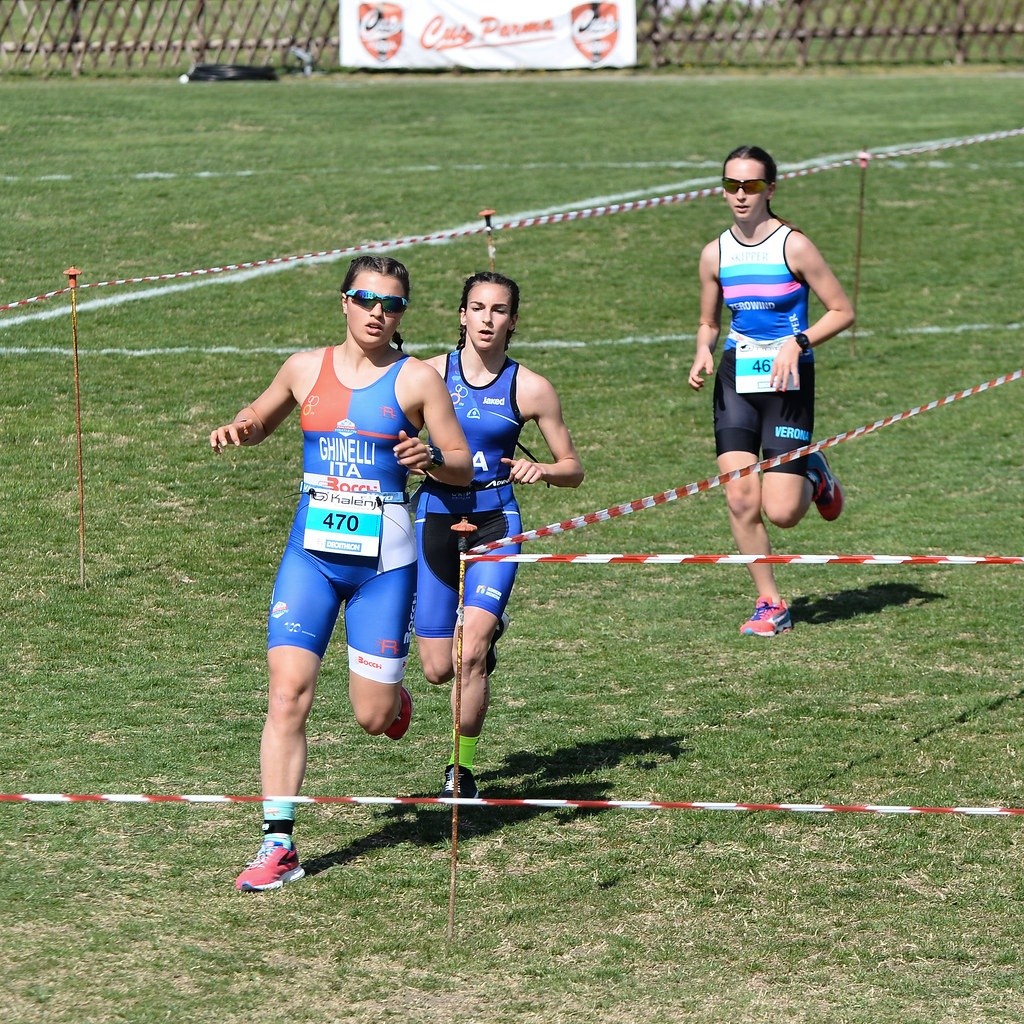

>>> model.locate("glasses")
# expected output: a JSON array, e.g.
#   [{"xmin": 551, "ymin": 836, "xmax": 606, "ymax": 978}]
[
  {"xmin": 345, "ymin": 289, "xmax": 409, "ymax": 314},
  {"xmin": 721, "ymin": 177, "xmax": 770, "ymax": 195}
]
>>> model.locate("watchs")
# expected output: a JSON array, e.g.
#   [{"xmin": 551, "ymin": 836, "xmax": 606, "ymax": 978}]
[
  {"xmin": 794, "ymin": 333, "xmax": 809, "ymax": 354},
  {"xmin": 420, "ymin": 445, "xmax": 444, "ymax": 475}
]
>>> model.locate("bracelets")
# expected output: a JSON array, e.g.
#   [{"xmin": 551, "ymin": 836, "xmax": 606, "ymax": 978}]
[{"xmin": 242, "ymin": 420, "xmax": 249, "ymax": 442}]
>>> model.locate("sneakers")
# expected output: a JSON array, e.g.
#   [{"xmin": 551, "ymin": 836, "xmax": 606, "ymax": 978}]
[
  {"xmin": 740, "ymin": 595, "xmax": 792, "ymax": 637},
  {"xmin": 486, "ymin": 612, "xmax": 510, "ymax": 678},
  {"xmin": 806, "ymin": 450, "xmax": 843, "ymax": 521},
  {"xmin": 384, "ymin": 685, "xmax": 412, "ymax": 740},
  {"xmin": 438, "ymin": 764, "xmax": 479, "ymax": 799},
  {"xmin": 235, "ymin": 840, "xmax": 305, "ymax": 891}
]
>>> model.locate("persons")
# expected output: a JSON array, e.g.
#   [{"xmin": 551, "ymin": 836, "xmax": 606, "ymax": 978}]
[
  {"xmin": 210, "ymin": 255, "xmax": 473, "ymax": 892},
  {"xmin": 415, "ymin": 272, "xmax": 584, "ymax": 800},
  {"xmin": 689, "ymin": 146, "xmax": 855, "ymax": 638}
]
